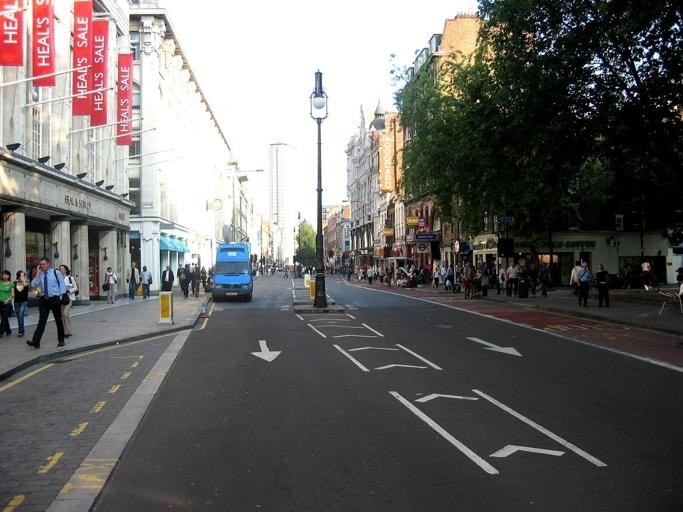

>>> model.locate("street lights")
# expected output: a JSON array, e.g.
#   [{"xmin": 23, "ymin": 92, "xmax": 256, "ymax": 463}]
[
  {"xmin": 310, "ymin": 64, "xmax": 332, "ymax": 305},
  {"xmin": 342, "ymin": 198, "xmax": 365, "ymax": 254},
  {"xmin": 293, "ymin": 213, "xmax": 303, "ymax": 278}
]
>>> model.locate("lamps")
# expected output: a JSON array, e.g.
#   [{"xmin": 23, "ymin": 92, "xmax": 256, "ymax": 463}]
[{"xmin": 4, "ymin": 139, "xmax": 131, "ymax": 200}]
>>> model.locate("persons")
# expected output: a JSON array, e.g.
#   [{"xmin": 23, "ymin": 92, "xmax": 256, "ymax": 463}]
[
  {"xmin": 139, "ymin": 265, "xmax": 152, "ymax": 300},
  {"xmin": 568, "ymin": 255, "xmax": 683, "ymax": 307},
  {"xmin": 126, "ymin": 261, "xmax": 139, "ymax": 302},
  {"xmin": 26, "ymin": 256, "xmax": 67, "ymax": 349},
  {"xmin": 162, "ymin": 265, "xmax": 174, "ymax": 291},
  {"xmin": 59, "ymin": 265, "xmax": 79, "ymax": 338},
  {"xmin": 325, "ymin": 259, "xmax": 554, "ymax": 300},
  {"xmin": 0, "ymin": 270, "xmax": 16, "ymax": 337},
  {"xmin": 14, "ymin": 270, "xmax": 29, "ymax": 337},
  {"xmin": 283, "ymin": 264, "xmax": 302, "ymax": 278},
  {"xmin": 177, "ymin": 262, "xmax": 212, "ymax": 300},
  {"xmin": 104, "ymin": 266, "xmax": 117, "ymax": 304}
]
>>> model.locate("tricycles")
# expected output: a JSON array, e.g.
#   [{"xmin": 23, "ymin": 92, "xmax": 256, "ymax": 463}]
[{"xmin": 460, "ymin": 278, "xmax": 481, "ymax": 299}]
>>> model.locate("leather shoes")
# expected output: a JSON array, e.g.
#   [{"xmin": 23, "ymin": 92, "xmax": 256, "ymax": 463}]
[
  {"xmin": 26, "ymin": 340, "xmax": 40, "ymax": 348},
  {"xmin": 57, "ymin": 341, "xmax": 65, "ymax": 347}
]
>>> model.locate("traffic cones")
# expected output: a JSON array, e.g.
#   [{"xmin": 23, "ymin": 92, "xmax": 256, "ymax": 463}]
[{"xmin": 200, "ymin": 304, "xmax": 209, "ymax": 318}]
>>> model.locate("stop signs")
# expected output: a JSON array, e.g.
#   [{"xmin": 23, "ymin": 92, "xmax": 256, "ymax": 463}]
[{"xmin": 418, "ymin": 243, "xmax": 426, "ymax": 251}]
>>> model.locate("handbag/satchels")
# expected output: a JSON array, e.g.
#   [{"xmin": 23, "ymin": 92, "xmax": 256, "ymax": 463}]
[
  {"xmin": 102, "ymin": 283, "xmax": 110, "ymax": 291},
  {"xmin": 61, "ymin": 293, "xmax": 70, "ymax": 306}
]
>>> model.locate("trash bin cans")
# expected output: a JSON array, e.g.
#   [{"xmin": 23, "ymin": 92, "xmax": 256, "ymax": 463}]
[{"xmin": 516, "ymin": 278, "xmax": 529, "ymax": 298}]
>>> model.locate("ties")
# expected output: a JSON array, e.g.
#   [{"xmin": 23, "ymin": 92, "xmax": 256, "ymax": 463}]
[{"xmin": 44, "ymin": 271, "xmax": 48, "ymax": 299}]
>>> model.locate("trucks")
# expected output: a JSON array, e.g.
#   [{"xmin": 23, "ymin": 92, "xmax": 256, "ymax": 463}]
[{"xmin": 211, "ymin": 242, "xmax": 254, "ymax": 305}]
